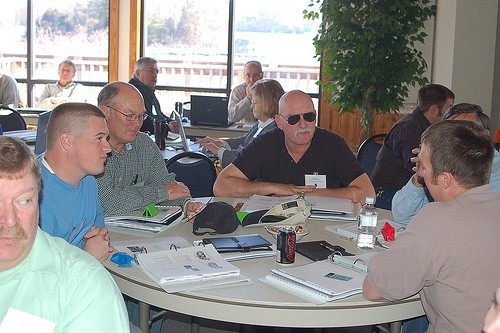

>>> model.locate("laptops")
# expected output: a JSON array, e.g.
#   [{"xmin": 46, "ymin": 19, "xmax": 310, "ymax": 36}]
[{"xmin": 189, "ymin": 95, "xmax": 229, "ymax": 126}]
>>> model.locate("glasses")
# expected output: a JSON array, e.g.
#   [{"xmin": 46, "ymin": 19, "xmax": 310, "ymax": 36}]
[
  {"xmin": 278, "ymin": 112, "xmax": 316, "ymax": 125},
  {"xmin": 107, "ymin": 106, "xmax": 148, "ymax": 122}
]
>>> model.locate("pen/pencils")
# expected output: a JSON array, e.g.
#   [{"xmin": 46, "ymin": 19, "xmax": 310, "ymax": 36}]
[
  {"xmin": 135, "ymin": 174, "xmax": 138, "ymax": 184},
  {"xmin": 318, "ymin": 242, "xmax": 335, "ymax": 252},
  {"xmin": 132, "ymin": 176, "xmax": 134, "ymax": 184}
]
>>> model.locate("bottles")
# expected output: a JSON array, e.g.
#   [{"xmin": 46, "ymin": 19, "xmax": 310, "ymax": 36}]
[
  {"xmin": 153, "ymin": 117, "xmax": 167, "ymax": 151},
  {"xmin": 175, "ymin": 102, "xmax": 182, "ymax": 114},
  {"xmin": 356, "ymin": 196, "xmax": 378, "ymax": 251}
]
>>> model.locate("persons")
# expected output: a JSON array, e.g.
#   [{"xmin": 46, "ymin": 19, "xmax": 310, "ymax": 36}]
[
  {"xmin": 361, "ymin": 121, "xmax": 500, "ymax": 333},
  {"xmin": 193, "ymin": 78, "xmax": 285, "ymax": 169},
  {"xmin": 0, "ymin": 74, "xmax": 27, "ymax": 108},
  {"xmin": 35, "ymin": 60, "xmax": 84, "ymax": 111},
  {"xmin": 370, "ymin": 84, "xmax": 455, "ymax": 211},
  {"xmin": 0, "ymin": 135, "xmax": 130, "ymax": 333},
  {"xmin": 482, "ymin": 288, "xmax": 500, "ymax": 333},
  {"xmin": 213, "ymin": 90, "xmax": 377, "ymax": 211},
  {"xmin": 127, "ymin": 56, "xmax": 179, "ymax": 135},
  {"xmin": 227, "ymin": 60, "xmax": 263, "ymax": 126},
  {"xmin": 94, "ymin": 82, "xmax": 202, "ymax": 221},
  {"xmin": 32, "ymin": 103, "xmax": 113, "ymax": 264},
  {"xmin": 392, "ymin": 103, "xmax": 500, "ymax": 225}
]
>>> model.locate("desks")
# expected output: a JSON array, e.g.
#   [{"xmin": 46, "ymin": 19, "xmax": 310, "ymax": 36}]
[
  {"xmin": 183, "ymin": 121, "xmax": 251, "ymax": 138},
  {"xmin": 101, "ymin": 196, "xmax": 426, "ymax": 333}
]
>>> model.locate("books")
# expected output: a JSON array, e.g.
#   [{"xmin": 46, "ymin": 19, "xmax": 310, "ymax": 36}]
[
  {"xmin": 239, "ymin": 193, "xmax": 359, "ymax": 222},
  {"xmin": 103, "ymin": 197, "xmax": 212, "ymax": 233},
  {"xmin": 107, "ymin": 234, "xmax": 252, "ymax": 293},
  {"xmin": 256, "ymin": 251, "xmax": 386, "ymax": 304},
  {"xmin": 322, "ymin": 219, "xmax": 408, "ymax": 249}
]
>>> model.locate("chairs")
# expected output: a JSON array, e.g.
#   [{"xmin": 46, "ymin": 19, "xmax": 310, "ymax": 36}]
[
  {"xmin": 166, "ymin": 151, "xmax": 217, "ymax": 198},
  {"xmin": 357, "ymin": 133, "xmax": 386, "ymax": 176},
  {"xmin": 0, "ymin": 105, "xmax": 26, "ymax": 132}
]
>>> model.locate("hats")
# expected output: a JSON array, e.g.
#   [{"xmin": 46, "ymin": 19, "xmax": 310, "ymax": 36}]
[
  {"xmin": 193, "ymin": 202, "xmax": 239, "ymax": 235},
  {"xmin": 242, "ymin": 199, "xmax": 312, "ymax": 229}
]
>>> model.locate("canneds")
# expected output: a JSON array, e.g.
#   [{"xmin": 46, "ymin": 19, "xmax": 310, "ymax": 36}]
[{"xmin": 276, "ymin": 227, "xmax": 296, "ymax": 264}]
